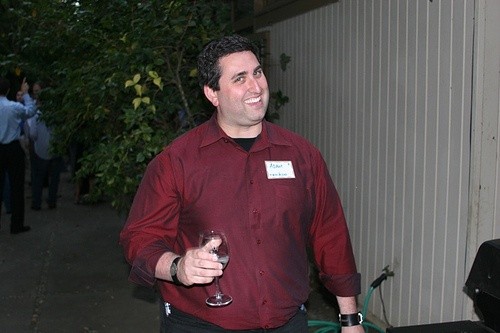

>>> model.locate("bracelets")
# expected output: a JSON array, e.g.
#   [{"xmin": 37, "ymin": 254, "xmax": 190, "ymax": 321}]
[{"xmin": 339, "ymin": 309, "xmax": 364, "ymax": 326}]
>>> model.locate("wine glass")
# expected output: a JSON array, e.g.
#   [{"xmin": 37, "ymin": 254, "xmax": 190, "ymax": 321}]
[{"xmin": 198, "ymin": 230, "xmax": 233, "ymax": 306}]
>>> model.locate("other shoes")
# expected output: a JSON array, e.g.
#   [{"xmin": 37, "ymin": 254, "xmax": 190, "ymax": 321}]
[
  {"xmin": 48, "ymin": 203, "xmax": 57, "ymax": 208},
  {"xmin": 31, "ymin": 205, "xmax": 42, "ymax": 210},
  {"xmin": 6, "ymin": 209, "xmax": 12, "ymax": 214},
  {"xmin": 10, "ymin": 224, "xmax": 32, "ymax": 235}
]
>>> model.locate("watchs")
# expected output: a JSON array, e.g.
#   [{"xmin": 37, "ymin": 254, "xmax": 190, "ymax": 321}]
[{"xmin": 169, "ymin": 256, "xmax": 183, "ymax": 286}]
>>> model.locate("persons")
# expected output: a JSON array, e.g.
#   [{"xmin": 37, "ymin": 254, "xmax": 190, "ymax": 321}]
[
  {"xmin": 0, "ymin": 81, "xmax": 97, "ymax": 215},
  {"xmin": 0, "ymin": 77, "xmax": 38, "ymax": 234},
  {"xmin": 118, "ymin": 33, "xmax": 366, "ymax": 332}
]
